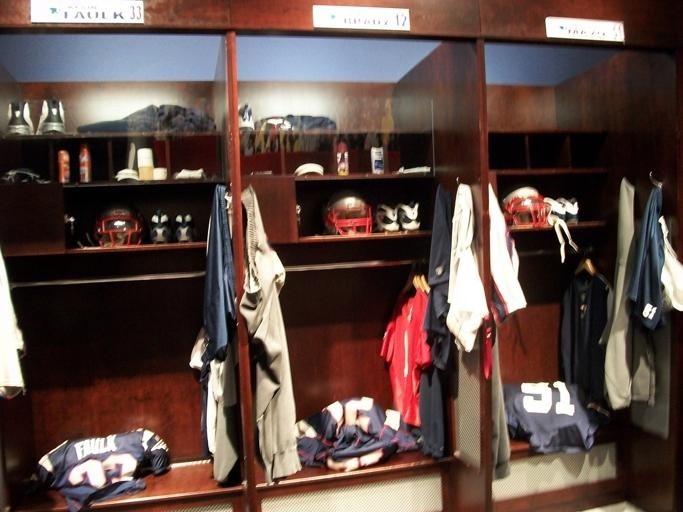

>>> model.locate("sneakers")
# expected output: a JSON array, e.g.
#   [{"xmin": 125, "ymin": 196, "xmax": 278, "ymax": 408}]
[
  {"xmin": 151, "ymin": 210, "xmax": 194, "ymax": 243},
  {"xmin": 373, "ymin": 201, "xmax": 420, "ymax": 232},
  {"xmin": 543, "ymin": 197, "xmax": 578, "ymax": 223},
  {"xmin": 7, "ymin": 102, "xmax": 34, "ymax": 135},
  {"xmin": 36, "ymin": 97, "xmax": 65, "ymax": 134}
]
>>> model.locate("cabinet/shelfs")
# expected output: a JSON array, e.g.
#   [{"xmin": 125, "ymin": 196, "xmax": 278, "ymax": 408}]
[{"xmin": 0, "ymin": 1, "xmax": 682, "ymax": 512}]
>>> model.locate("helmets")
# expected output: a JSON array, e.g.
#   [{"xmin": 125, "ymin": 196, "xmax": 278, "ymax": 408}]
[
  {"xmin": 94, "ymin": 205, "xmax": 144, "ymax": 246},
  {"xmin": 323, "ymin": 188, "xmax": 373, "ymax": 235},
  {"xmin": 503, "ymin": 186, "xmax": 547, "ymax": 229}
]
